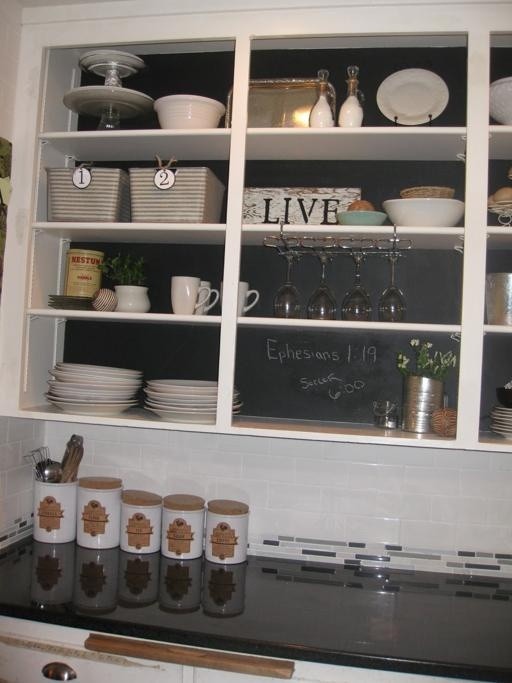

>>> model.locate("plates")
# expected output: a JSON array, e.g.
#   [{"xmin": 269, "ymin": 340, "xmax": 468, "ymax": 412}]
[
  {"xmin": 45, "ymin": 362, "xmax": 245, "ymax": 422},
  {"xmin": 376, "ymin": 68, "xmax": 449, "ymax": 125},
  {"xmin": 489, "ymin": 406, "xmax": 512, "ymax": 438}
]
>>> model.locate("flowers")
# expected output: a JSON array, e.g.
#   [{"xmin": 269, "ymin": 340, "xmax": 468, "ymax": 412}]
[
  {"xmin": 5, "ymin": 4, "xmax": 512, "ymax": 451},
  {"xmin": 392, "ymin": 333, "xmax": 458, "ymax": 377}
]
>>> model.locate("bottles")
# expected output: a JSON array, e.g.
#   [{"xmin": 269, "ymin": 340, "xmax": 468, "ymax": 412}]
[
  {"xmin": 311, "ymin": 69, "xmax": 335, "ymax": 127},
  {"xmin": 376, "ymin": 401, "xmax": 398, "ymax": 429},
  {"xmin": 338, "ymin": 65, "xmax": 366, "ymax": 127}
]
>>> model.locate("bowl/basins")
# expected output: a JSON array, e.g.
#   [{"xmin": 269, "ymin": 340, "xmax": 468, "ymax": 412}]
[
  {"xmin": 496, "ymin": 387, "xmax": 512, "ymax": 408},
  {"xmin": 335, "ymin": 211, "xmax": 387, "ymax": 226},
  {"xmin": 153, "ymin": 94, "xmax": 227, "ymax": 129},
  {"xmin": 385, "ymin": 198, "xmax": 464, "ymax": 227}
]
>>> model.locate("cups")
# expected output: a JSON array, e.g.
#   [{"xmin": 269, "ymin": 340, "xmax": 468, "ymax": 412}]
[
  {"xmin": 238, "ymin": 281, "xmax": 260, "ymax": 316},
  {"xmin": 169, "ymin": 276, "xmax": 217, "ymax": 314},
  {"xmin": 28, "ymin": 476, "xmax": 248, "ymax": 564}
]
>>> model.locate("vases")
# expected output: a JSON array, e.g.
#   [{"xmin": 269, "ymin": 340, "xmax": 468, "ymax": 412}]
[{"xmin": 401, "ymin": 376, "xmax": 443, "ymax": 434}]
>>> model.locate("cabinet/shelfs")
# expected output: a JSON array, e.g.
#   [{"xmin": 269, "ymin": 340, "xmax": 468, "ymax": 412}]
[{"xmin": 0, "ymin": 618, "xmax": 468, "ymax": 683}]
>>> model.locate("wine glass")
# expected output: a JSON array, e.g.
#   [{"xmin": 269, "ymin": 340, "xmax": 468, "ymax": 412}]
[{"xmin": 276, "ymin": 251, "xmax": 406, "ymax": 323}]
